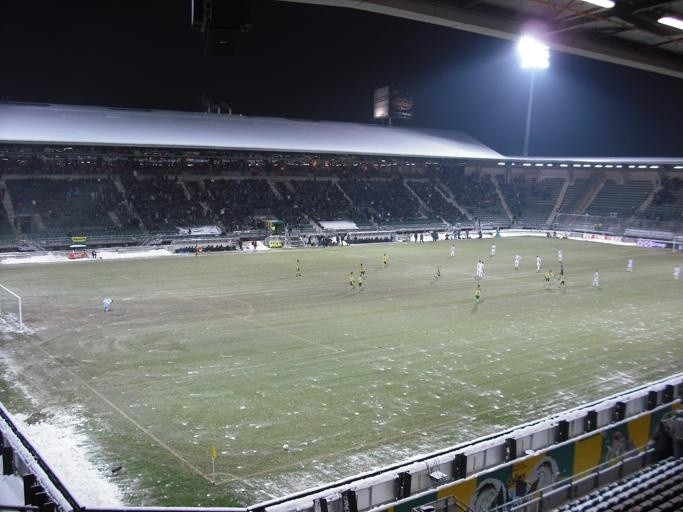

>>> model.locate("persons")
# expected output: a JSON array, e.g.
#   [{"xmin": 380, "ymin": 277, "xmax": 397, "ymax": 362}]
[
  {"xmin": 448, "ymin": 245, "xmax": 455, "ymax": 257},
  {"xmin": 558, "ymin": 274, "xmax": 565, "ymax": 288},
  {"xmin": 357, "ymin": 274, "xmax": 362, "ymax": 290},
  {"xmin": 544, "ymin": 269, "xmax": 554, "ymax": 288},
  {"xmin": 513, "ymin": 253, "xmax": 520, "ymax": 270},
  {"xmin": 673, "ymin": 266, "xmax": 679, "ymax": 279},
  {"xmin": 558, "ymin": 249, "xmax": 562, "ymax": 261},
  {"xmin": 102, "ymin": 299, "xmax": 112, "ymax": 313},
  {"xmin": 557, "ymin": 261, "xmax": 564, "ymax": 275},
  {"xmin": 591, "ymin": 270, "xmax": 599, "ymax": 288},
  {"xmin": 490, "ymin": 244, "xmax": 495, "ymax": 256},
  {"xmin": 651, "ymin": 174, "xmax": 681, "ymax": 205},
  {"xmin": 474, "ymin": 284, "xmax": 481, "ymax": 303},
  {"xmin": 476, "ymin": 260, "xmax": 481, "ymax": 277},
  {"xmin": 92, "ymin": 250, "xmax": 96, "ymax": 258},
  {"xmin": 383, "ymin": 253, "xmax": 387, "ymax": 267},
  {"xmin": 296, "ymin": 260, "xmax": 303, "ymax": 279},
  {"xmin": 348, "ymin": 272, "xmax": 354, "ymax": 288},
  {"xmin": 359, "ymin": 263, "xmax": 365, "ymax": 280},
  {"xmin": 626, "ymin": 258, "xmax": 632, "ymax": 272},
  {"xmin": 553, "ymin": 204, "xmax": 663, "ymax": 239},
  {"xmin": 479, "ymin": 262, "xmax": 485, "ymax": 276},
  {"xmin": 0, "ymin": 149, "xmax": 552, "ymax": 253},
  {"xmin": 535, "ymin": 256, "xmax": 543, "ymax": 272}
]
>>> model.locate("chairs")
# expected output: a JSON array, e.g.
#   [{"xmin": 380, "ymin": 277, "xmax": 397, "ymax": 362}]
[{"xmin": 549, "ymin": 455, "xmax": 683, "ymax": 512}]
